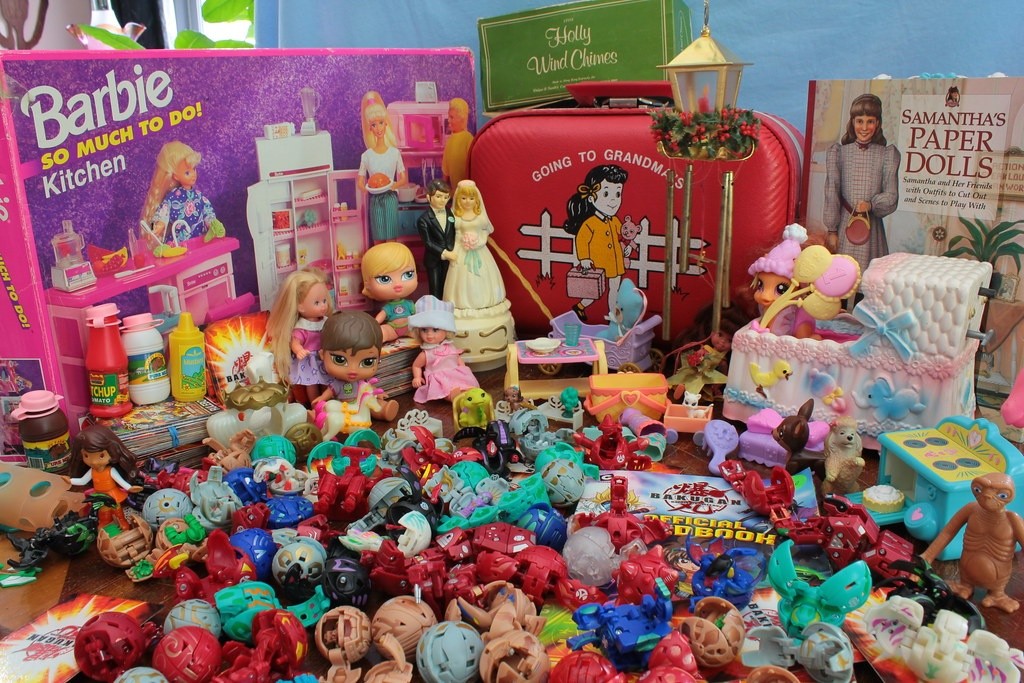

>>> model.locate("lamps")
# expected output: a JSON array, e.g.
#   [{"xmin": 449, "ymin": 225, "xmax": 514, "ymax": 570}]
[{"xmin": 656, "ymin": 0, "xmax": 762, "ymax": 341}]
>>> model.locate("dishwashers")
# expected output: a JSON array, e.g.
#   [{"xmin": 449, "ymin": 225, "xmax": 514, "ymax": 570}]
[{"xmin": 174, "ymin": 251, "xmax": 237, "ymax": 326}]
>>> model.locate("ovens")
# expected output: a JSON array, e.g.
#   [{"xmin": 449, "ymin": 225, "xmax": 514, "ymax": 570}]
[{"xmin": 844, "ymin": 449, "xmax": 1024, "ymax": 561}]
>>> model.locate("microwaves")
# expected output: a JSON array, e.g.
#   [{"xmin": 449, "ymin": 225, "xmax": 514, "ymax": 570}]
[{"xmin": 385, "ymin": 100, "xmax": 453, "ymax": 149}]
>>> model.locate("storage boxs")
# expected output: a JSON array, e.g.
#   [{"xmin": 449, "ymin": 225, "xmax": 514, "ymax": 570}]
[{"xmin": 478, "ymin": 0, "xmax": 692, "ymax": 112}]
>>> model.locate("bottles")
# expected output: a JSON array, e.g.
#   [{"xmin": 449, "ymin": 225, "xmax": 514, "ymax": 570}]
[
  {"xmin": 119, "ymin": 313, "xmax": 170, "ymax": 407},
  {"xmin": 85, "ymin": 302, "xmax": 133, "ymax": 417},
  {"xmin": 11, "ymin": 389, "xmax": 75, "ymax": 476},
  {"xmin": 168, "ymin": 310, "xmax": 206, "ymax": 401},
  {"xmin": 128, "ymin": 227, "xmax": 140, "ymax": 262}
]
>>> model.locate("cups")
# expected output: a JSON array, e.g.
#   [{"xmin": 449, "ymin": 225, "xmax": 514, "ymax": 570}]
[
  {"xmin": 564, "ymin": 322, "xmax": 582, "ymax": 346},
  {"xmin": 276, "ymin": 242, "xmax": 291, "ymax": 266}
]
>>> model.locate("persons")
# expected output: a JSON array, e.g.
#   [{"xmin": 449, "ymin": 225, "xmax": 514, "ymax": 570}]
[
  {"xmin": 61, "ymin": 425, "xmax": 143, "ymax": 509},
  {"xmin": 307, "ymin": 310, "xmax": 400, "ymax": 425},
  {"xmin": 266, "ymin": 270, "xmax": 332, "ymax": 409},
  {"xmin": 664, "ymin": 307, "xmax": 753, "ymax": 403},
  {"xmin": 748, "ymin": 223, "xmax": 823, "ymax": 341},
  {"xmin": 417, "ymin": 180, "xmax": 508, "ymax": 309},
  {"xmin": 361, "ymin": 241, "xmax": 418, "ymax": 344},
  {"xmin": 407, "ymin": 295, "xmax": 480, "ymax": 405}
]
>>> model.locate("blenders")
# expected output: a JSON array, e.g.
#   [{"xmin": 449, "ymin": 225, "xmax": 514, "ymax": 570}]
[
  {"xmin": 300, "ymin": 85, "xmax": 321, "ymax": 135},
  {"xmin": 48, "ymin": 220, "xmax": 98, "ymax": 293}
]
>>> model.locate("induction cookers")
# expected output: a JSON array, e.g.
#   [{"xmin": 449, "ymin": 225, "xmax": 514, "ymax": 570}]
[{"xmin": 874, "ymin": 405, "xmax": 1024, "ymax": 492}]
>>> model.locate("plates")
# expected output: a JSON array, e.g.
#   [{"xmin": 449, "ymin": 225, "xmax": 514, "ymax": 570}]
[
  {"xmin": 525, "ymin": 337, "xmax": 561, "ymax": 353},
  {"xmin": 365, "ymin": 179, "xmax": 392, "ymax": 194}
]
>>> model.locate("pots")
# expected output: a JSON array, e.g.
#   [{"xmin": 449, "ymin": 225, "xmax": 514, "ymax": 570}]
[{"xmin": 395, "ymin": 183, "xmax": 421, "ymax": 202}]
